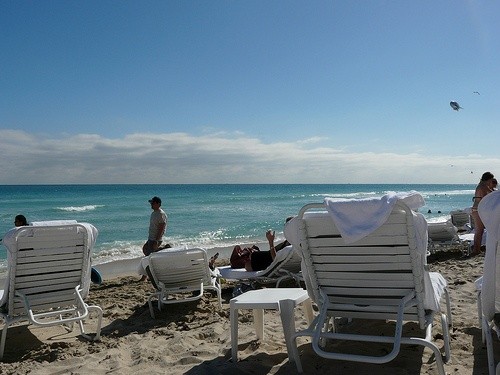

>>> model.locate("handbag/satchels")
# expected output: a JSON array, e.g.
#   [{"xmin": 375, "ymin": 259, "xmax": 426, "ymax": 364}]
[{"xmin": 231, "ymin": 245, "xmax": 260, "ymax": 266}]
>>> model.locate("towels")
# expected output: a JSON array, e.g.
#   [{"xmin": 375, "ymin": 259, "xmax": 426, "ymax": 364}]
[{"xmin": 324, "ymin": 190, "xmax": 447, "ymax": 312}]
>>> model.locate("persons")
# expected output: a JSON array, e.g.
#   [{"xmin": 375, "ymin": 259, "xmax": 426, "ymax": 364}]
[
  {"xmin": 230, "ymin": 217, "xmax": 294, "ymax": 272},
  {"xmin": 136, "ymin": 197, "xmax": 168, "ymax": 282},
  {"xmin": 471, "ymin": 172, "xmax": 498, "ymax": 254},
  {"xmin": 13, "ymin": 215, "xmax": 29, "ymax": 227}
]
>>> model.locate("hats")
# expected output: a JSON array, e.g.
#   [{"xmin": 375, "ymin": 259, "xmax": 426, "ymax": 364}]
[{"xmin": 148, "ymin": 197, "xmax": 161, "ymax": 203}]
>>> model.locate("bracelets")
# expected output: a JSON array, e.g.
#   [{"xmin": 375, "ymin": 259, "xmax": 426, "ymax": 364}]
[{"xmin": 271, "ymin": 247, "xmax": 274, "ymax": 249}]
